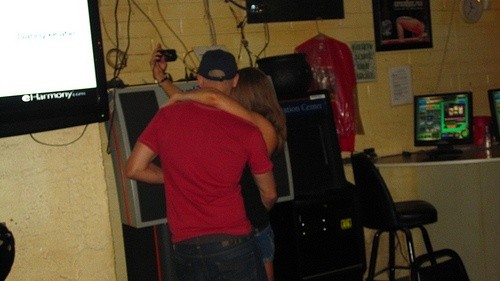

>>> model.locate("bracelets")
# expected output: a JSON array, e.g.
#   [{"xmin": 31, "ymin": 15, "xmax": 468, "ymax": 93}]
[{"xmin": 158, "ymin": 76, "xmax": 169, "ymax": 87}]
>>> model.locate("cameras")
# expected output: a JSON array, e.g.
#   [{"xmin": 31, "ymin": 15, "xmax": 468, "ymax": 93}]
[{"xmin": 157, "ymin": 49, "xmax": 177, "ymax": 61}]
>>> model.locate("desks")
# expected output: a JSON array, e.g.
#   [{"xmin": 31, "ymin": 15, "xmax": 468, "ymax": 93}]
[{"xmin": 374, "ymin": 150, "xmax": 500, "ymax": 281}]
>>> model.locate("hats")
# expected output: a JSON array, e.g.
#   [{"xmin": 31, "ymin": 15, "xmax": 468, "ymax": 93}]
[{"xmin": 197, "ymin": 49, "xmax": 238, "ymax": 81}]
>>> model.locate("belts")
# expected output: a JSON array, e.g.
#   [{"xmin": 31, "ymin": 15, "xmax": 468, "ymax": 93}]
[{"xmin": 174, "ymin": 233, "xmax": 254, "ymax": 246}]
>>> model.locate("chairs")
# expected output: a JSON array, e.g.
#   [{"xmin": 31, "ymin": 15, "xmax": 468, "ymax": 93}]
[
  {"xmin": 351, "ymin": 153, "xmax": 439, "ymax": 281},
  {"xmin": 410, "ymin": 248, "xmax": 470, "ymax": 281}
]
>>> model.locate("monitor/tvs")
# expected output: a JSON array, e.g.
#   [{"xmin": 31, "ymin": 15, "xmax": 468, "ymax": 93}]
[
  {"xmin": 246, "ymin": 0, "xmax": 345, "ymax": 24},
  {"xmin": 414, "ymin": 91, "xmax": 474, "ymax": 146},
  {"xmin": 0, "ymin": 0, "xmax": 109, "ymax": 139},
  {"xmin": 488, "ymin": 88, "xmax": 500, "ymax": 141}
]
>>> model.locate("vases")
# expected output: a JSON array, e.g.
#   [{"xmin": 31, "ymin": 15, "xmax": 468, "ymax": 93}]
[{"xmin": 255, "ymin": 52, "xmax": 315, "ymax": 99}]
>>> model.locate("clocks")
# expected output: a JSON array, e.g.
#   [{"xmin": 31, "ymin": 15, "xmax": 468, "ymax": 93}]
[{"xmin": 459, "ymin": 0, "xmax": 485, "ymax": 24}]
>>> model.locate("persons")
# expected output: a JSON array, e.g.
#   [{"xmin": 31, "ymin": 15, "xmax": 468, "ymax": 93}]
[
  {"xmin": 148, "ymin": 43, "xmax": 287, "ymax": 281},
  {"xmin": 124, "ymin": 48, "xmax": 277, "ymax": 281}
]
